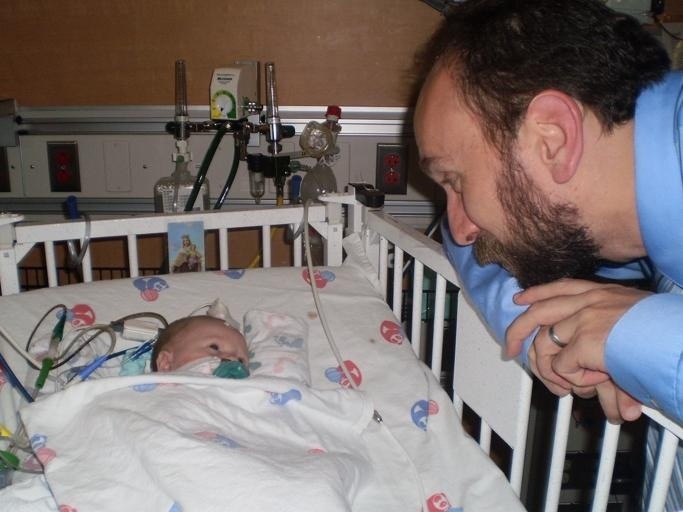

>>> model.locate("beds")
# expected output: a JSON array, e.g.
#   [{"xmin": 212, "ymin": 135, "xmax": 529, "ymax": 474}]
[{"xmin": 0, "ymin": 187, "xmax": 683, "ymax": 512}]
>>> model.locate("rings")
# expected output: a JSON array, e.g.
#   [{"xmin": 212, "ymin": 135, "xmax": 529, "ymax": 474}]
[{"xmin": 549, "ymin": 325, "xmax": 567, "ymax": 348}]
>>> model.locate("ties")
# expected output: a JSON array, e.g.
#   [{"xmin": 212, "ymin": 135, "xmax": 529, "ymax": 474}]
[{"xmin": 640, "ymin": 271, "xmax": 683, "ymax": 512}]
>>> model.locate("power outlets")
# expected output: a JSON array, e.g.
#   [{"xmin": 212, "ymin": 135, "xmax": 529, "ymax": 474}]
[{"xmin": 375, "ymin": 143, "xmax": 410, "ymax": 195}]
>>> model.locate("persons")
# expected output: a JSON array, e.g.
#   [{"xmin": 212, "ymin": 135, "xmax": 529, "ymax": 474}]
[
  {"xmin": 173, "ymin": 234, "xmax": 203, "ymax": 273},
  {"xmin": 145, "ymin": 315, "xmax": 251, "ymax": 381},
  {"xmin": 184, "ymin": 244, "xmax": 201, "ymax": 270},
  {"xmin": 398, "ymin": 0, "xmax": 683, "ymax": 437}
]
